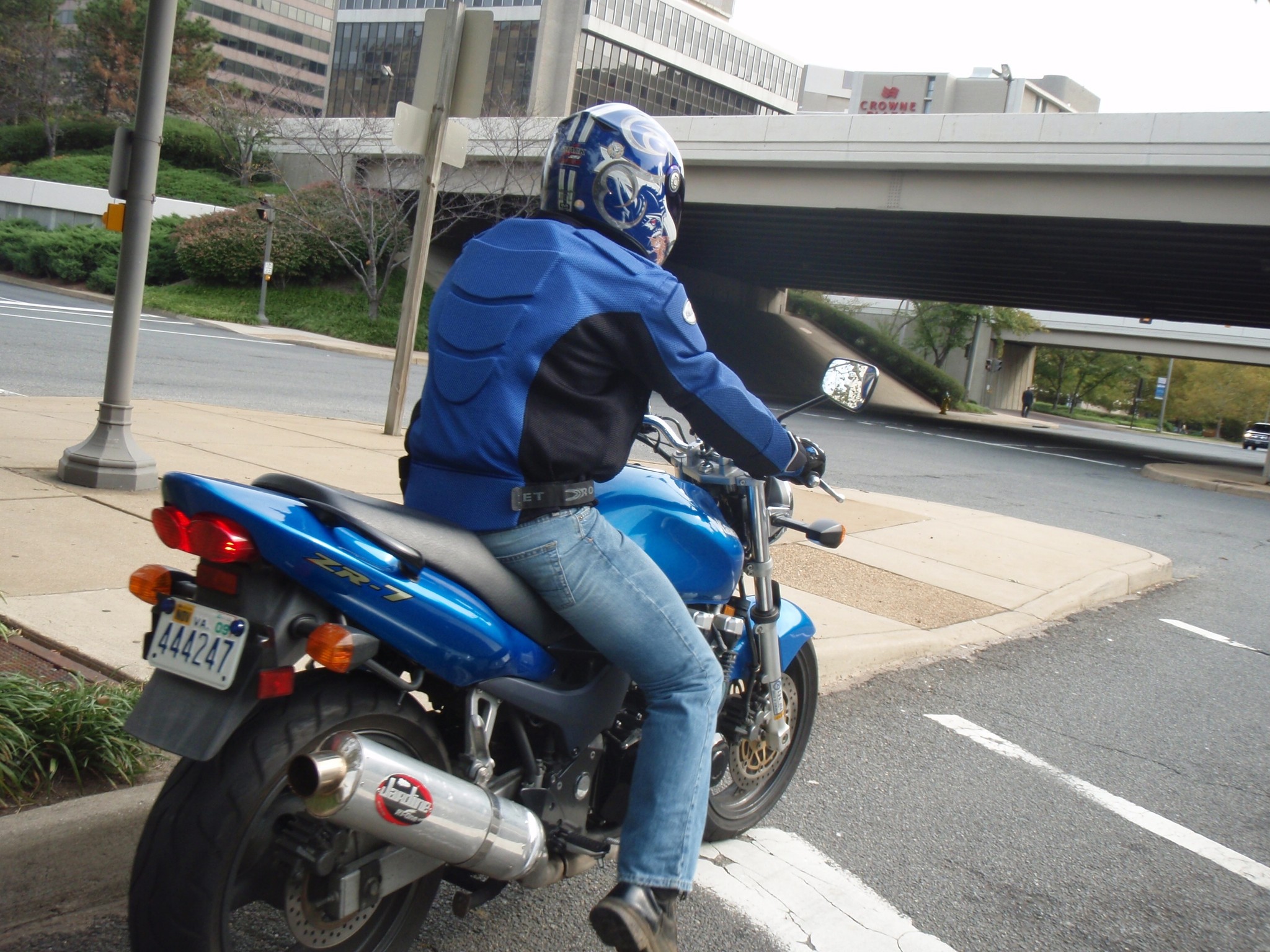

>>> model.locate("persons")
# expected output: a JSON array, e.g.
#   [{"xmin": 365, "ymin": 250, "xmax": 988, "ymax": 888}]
[
  {"xmin": 396, "ymin": 104, "xmax": 830, "ymax": 952},
  {"xmin": 1021, "ymin": 387, "xmax": 1033, "ymax": 418}
]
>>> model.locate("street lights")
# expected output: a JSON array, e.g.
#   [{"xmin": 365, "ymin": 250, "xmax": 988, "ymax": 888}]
[{"xmin": 380, "ymin": 65, "xmax": 394, "ymax": 118}]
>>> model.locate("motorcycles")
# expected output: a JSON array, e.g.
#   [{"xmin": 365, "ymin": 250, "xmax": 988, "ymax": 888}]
[{"xmin": 119, "ymin": 353, "xmax": 880, "ymax": 952}]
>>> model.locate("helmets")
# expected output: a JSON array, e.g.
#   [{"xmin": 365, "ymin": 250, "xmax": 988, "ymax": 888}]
[{"xmin": 539, "ymin": 102, "xmax": 685, "ymax": 266}]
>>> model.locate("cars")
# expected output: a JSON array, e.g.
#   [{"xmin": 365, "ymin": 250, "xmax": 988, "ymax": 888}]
[{"xmin": 1242, "ymin": 423, "xmax": 1270, "ymax": 450}]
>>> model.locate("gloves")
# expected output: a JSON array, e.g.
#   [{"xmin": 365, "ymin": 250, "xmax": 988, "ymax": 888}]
[{"xmin": 785, "ymin": 429, "xmax": 827, "ymax": 487}]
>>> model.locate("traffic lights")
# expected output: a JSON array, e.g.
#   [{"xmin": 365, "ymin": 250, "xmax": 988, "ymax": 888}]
[
  {"xmin": 994, "ymin": 359, "xmax": 1003, "ymax": 371},
  {"xmin": 986, "ymin": 359, "xmax": 992, "ymax": 371},
  {"xmin": 255, "ymin": 208, "xmax": 272, "ymax": 223}
]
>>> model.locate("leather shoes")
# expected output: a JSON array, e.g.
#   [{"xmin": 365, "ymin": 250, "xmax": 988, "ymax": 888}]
[{"xmin": 588, "ymin": 882, "xmax": 680, "ymax": 952}]
